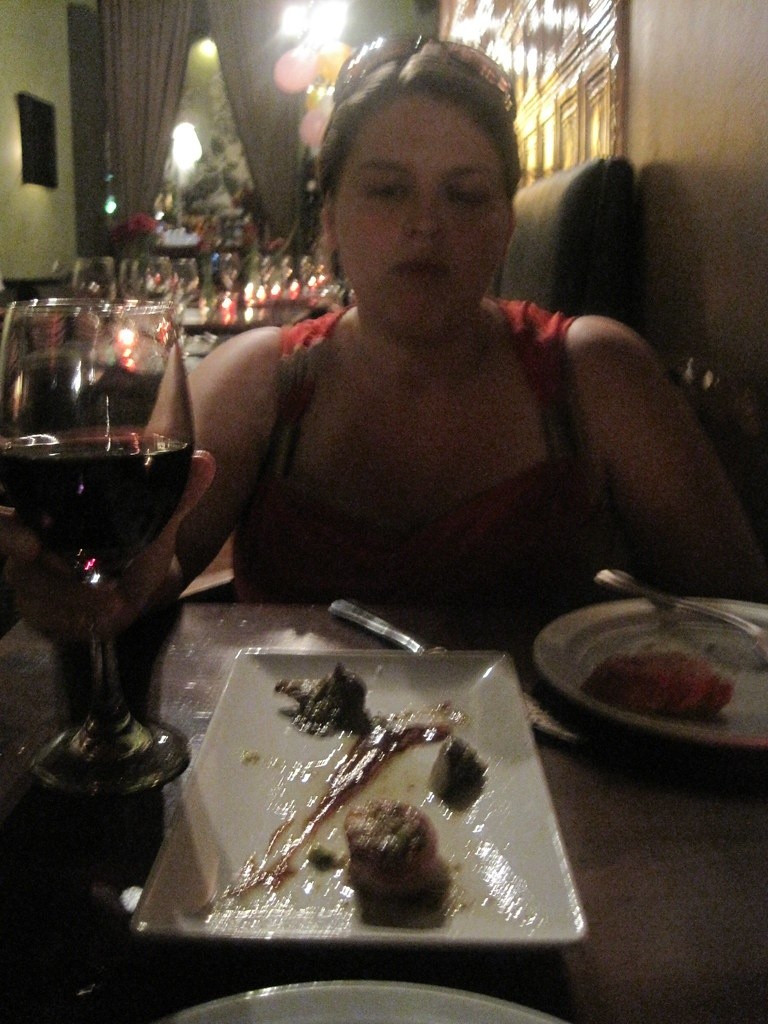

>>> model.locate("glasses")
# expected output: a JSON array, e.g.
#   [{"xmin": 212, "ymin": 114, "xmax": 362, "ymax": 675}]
[{"xmin": 321, "ymin": 35, "xmax": 517, "ymax": 150}]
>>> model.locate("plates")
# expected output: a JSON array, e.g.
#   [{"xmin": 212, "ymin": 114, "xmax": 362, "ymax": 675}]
[
  {"xmin": 534, "ymin": 594, "xmax": 767, "ymax": 746},
  {"xmin": 132, "ymin": 650, "xmax": 588, "ymax": 945},
  {"xmin": 154, "ymin": 978, "xmax": 568, "ymax": 1024}
]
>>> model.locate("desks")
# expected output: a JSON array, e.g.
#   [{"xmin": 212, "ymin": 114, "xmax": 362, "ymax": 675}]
[{"xmin": 180, "ymin": 305, "xmax": 318, "ymax": 336}]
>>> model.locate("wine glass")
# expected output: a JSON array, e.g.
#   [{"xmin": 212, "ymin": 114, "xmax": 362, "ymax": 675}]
[
  {"xmin": 1, "ymin": 296, "xmax": 193, "ymax": 791},
  {"xmin": 70, "ymin": 256, "xmax": 202, "ymax": 313}
]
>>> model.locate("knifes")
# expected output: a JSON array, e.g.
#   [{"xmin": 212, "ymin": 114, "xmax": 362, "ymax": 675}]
[{"xmin": 328, "ymin": 599, "xmax": 579, "ymax": 743}]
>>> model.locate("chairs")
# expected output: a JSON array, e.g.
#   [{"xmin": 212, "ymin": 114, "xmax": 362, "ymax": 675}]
[{"xmin": 482, "ymin": 155, "xmax": 637, "ymax": 321}]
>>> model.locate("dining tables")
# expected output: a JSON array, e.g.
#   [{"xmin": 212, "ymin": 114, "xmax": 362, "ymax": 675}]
[{"xmin": 0, "ymin": 604, "xmax": 768, "ymax": 1024}]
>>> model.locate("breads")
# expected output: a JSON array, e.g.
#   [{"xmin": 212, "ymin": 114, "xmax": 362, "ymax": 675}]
[{"xmin": 581, "ymin": 651, "xmax": 734, "ymax": 717}]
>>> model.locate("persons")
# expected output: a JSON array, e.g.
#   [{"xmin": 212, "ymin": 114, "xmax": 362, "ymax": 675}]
[{"xmin": 3, "ymin": 43, "xmax": 766, "ymax": 641}]
